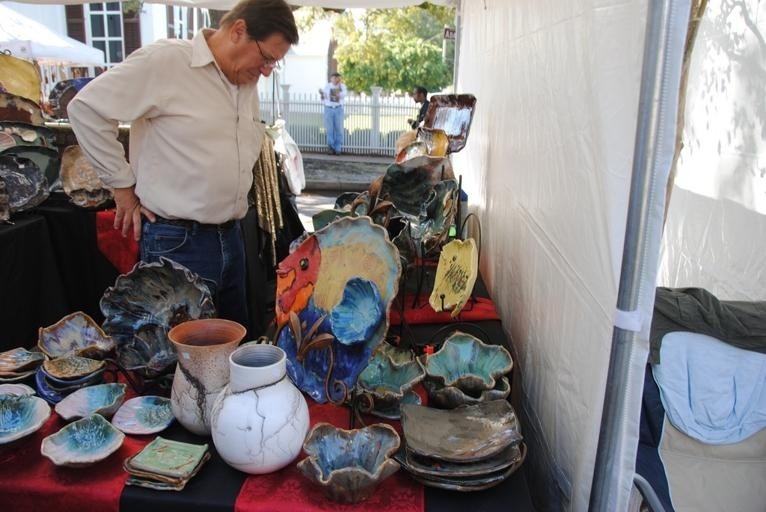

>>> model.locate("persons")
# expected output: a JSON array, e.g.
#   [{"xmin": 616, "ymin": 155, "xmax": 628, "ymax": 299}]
[
  {"xmin": 407, "ymin": 85, "xmax": 430, "ymax": 128},
  {"xmin": 319, "ymin": 72, "xmax": 347, "ymax": 154},
  {"xmin": 68, "ymin": 0, "xmax": 299, "ymax": 327}
]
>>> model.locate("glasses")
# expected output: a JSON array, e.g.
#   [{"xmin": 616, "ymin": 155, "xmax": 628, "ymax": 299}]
[{"xmin": 256, "ymin": 40, "xmax": 281, "ymax": 70}]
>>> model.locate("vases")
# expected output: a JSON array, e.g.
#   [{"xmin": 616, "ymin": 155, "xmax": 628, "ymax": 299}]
[
  {"xmin": 168, "ymin": 318, "xmax": 246, "ymax": 434},
  {"xmin": 210, "ymin": 343, "xmax": 310, "ymax": 473}
]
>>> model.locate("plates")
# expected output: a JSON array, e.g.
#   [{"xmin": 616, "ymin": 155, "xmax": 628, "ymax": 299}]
[
  {"xmin": 130, "ymin": 436, "xmax": 209, "ymax": 479},
  {"xmin": 428, "ymin": 238, "xmax": 477, "ymax": 316},
  {"xmin": 392, "ymin": 443, "xmax": 529, "ymax": 493},
  {"xmin": 122, "ymin": 451, "xmax": 213, "ymax": 485},
  {"xmin": 402, "ymin": 444, "xmax": 522, "ymax": 476},
  {"xmin": 401, "ymin": 401, "xmax": 523, "ymax": 462},
  {"xmin": 0, "ymin": 348, "xmax": 45, "ymax": 372},
  {"xmin": 423, "ymin": 94, "xmax": 476, "ymax": 152},
  {"xmin": 113, "ymin": 396, "xmax": 175, "ymax": 431},
  {"xmin": 37, "ymin": 311, "xmax": 116, "ymax": 367},
  {"xmin": 2, "ymin": 372, "xmax": 34, "ymax": 382},
  {"xmin": 415, "ymin": 129, "xmax": 448, "ymax": 162},
  {"xmin": 127, "ymin": 477, "xmax": 188, "ymax": 492},
  {"xmin": 0, "ymin": 370, "xmax": 24, "ymax": 377}
]
